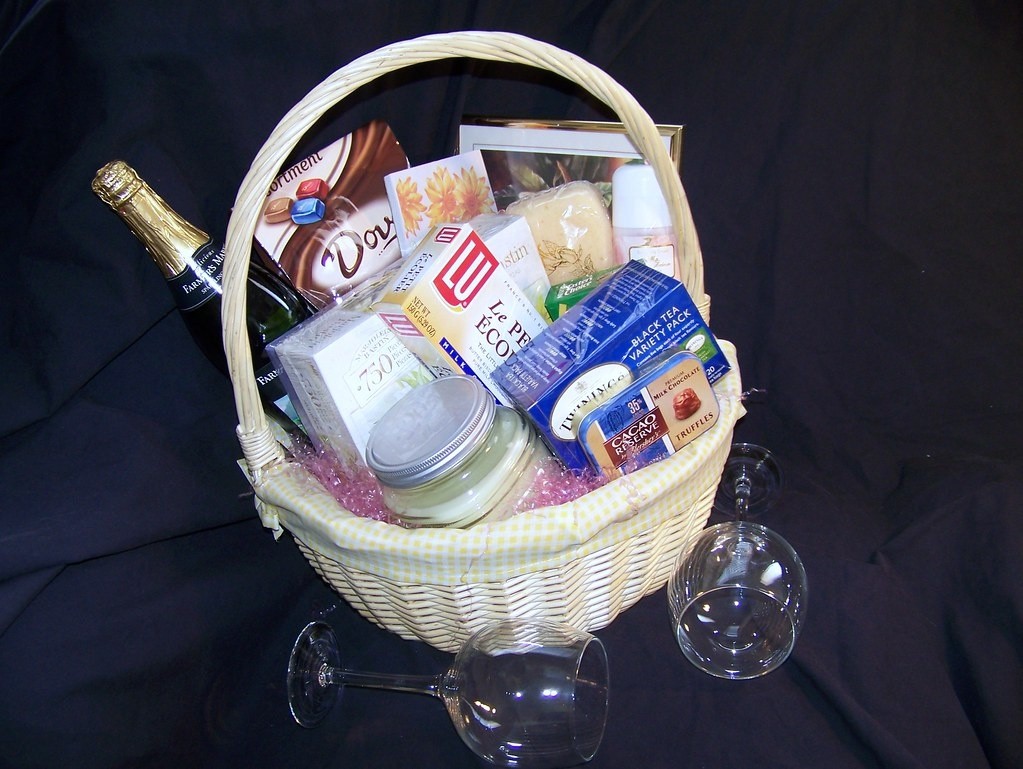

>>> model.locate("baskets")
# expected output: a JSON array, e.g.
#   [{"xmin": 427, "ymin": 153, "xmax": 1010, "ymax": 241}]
[{"xmin": 218, "ymin": 32, "xmax": 746, "ymax": 660}]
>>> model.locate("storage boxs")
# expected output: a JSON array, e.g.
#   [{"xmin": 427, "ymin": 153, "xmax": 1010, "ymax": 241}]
[{"xmin": 232, "ymin": 115, "xmax": 729, "ymax": 484}]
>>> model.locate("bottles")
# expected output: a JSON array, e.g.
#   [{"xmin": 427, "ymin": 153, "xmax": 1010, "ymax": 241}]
[
  {"xmin": 364, "ymin": 374, "xmax": 561, "ymax": 529},
  {"xmin": 91, "ymin": 159, "xmax": 315, "ymax": 449}
]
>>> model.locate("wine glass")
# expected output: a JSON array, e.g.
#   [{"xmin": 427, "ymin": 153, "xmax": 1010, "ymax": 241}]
[
  {"xmin": 665, "ymin": 441, "xmax": 809, "ymax": 679},
  {"xmin": 286, "ymin": 620, "xmax": 611, "ymax": 769}
]
